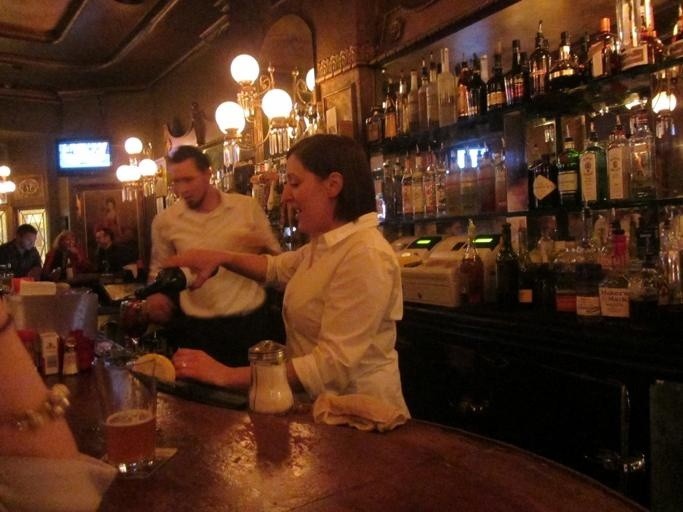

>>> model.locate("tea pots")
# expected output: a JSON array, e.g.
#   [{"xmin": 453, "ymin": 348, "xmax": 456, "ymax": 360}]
[{"xmin": 251, "ymin": 11, "xmax": 324, "ymax": 163}]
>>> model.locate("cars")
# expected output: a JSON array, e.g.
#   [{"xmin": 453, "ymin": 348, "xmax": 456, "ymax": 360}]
[
  {"xmin": 16, "ymin": 327, "xmax": 38, "ymax": 370},
  {"xmin": 94, "ymin": 353, "xmax": 157, "ymax": 473}
]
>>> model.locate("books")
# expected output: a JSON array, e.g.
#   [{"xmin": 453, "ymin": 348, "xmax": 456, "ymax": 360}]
[{"xmin": 0, "ymin": 288, "xmax": 99, "ymax": 349}]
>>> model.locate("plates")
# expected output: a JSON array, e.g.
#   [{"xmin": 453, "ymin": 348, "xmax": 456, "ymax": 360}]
[
  {"xmin": 361, "ymin": 0, "xmax": 683, "ymax": 339},
  {"xmin": 395, "ymin": 313, "xmax": 523, "ymax": 447},
  {"xmin": 521, "ymin": 333, "xmax": 652, "ymax": 502}
]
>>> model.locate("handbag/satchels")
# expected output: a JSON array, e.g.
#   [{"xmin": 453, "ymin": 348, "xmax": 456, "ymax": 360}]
[
  {"xmin": 0, "ymin": 310, "xmax": 11, "ymax": 335},
  {"xmin": 0, "ymin": 384, "xmax": 72, "ymax": 432}
]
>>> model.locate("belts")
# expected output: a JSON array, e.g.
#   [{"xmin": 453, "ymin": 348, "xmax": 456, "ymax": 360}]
[{"xmin": 120, "ymin": 299, "xmax": 149, "ymax": 366}]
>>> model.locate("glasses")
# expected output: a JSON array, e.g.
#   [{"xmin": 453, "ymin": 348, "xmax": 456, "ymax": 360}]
[
  {"xmin": 285, "ymin": 62, "xmax": 320, "ymax": 146},
  {"xmin": 0, "ymin": 164, "xmax": 19, "ymax": 210},
  {"xmin": 113, "ymin": 131, "xmax": 158, "ymax": 202},
  {"xmin": 210, "ymin": 51, "xmax": 296, "ymax": 164}
]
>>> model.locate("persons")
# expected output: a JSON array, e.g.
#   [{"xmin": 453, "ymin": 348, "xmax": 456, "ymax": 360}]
[
  {"xmin": 42, "ymin": 228, "xmax": 84, "ymax": 280},
  {"xmin": 162, "ymin": 132, "xmax": 413, "ymax": 420},
  {"xmin": 144, "ymin": 145, "xmax": 284, "ymax": 368},
  {"xmin": 0, "ymin": 289, "xmax": 117, "ymax": 512},
  {"xmin": 86, "ymin": 226, "xmax": 134, "ymax": 273},
  {"xmin": 0, "ymin": 223, "xmax": 42, "ymax": 281},
  {"xmin": 98, "ymin": 198, "xmax": 126, "ymax": 242}
]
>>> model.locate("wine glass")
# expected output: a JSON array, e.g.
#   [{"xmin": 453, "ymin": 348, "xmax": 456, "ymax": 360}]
[{"xmin": 181, "ymin": 361, "xmax": 187, "ymax": 367}]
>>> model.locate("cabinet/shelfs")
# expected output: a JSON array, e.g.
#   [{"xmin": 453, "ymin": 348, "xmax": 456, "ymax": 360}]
[
  {"xmin": 497, "ymin": 220, "xmax": 667, "ymax": 319},
  {"xmin": 61, "ymin": 338, "xmax": 78, "ymax": 376},
  {"xmin": 461, "ymin": 220, "xmax": 485, "ymax": 311},
  {"xmin": 268, "ymin": 106, "xmax": 320, "ymax": 157},
  {"xmin": 133, "ymin": 263, "xmax": 220, "ymax": 300},
  {"xmin": 247, "ymin": 339, "xmax": 295, "ymax": 415},
  {"xmin": 265, "ymin": 180, "xmax": 293, "ymax": 229},
  {"xmin": 214, "ymin": 165, "xmax": 234, "ymax": 194}
]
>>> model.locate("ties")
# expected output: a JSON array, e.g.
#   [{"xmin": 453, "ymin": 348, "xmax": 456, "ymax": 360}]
[{"xmin": 55, "ymin": 138, "xmax": 112, "ymax": 172}]
